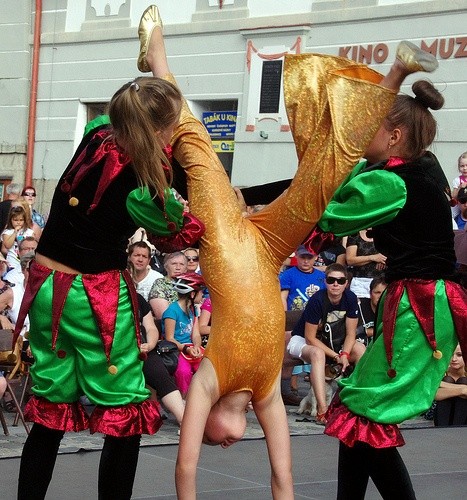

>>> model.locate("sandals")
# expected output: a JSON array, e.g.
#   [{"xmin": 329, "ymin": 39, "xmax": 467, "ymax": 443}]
[
  {"xmin": 3, "ymin": 399, "xmax": 18, "ymax": 413},
  {"xmin": 316, "ymin": 414, "xmax": 328, "ymax": 425}
]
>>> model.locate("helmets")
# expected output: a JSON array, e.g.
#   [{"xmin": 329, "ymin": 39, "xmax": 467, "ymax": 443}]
[{"xmin": 170, "ymin": 272, "xmax": 207, "ymax": 294}]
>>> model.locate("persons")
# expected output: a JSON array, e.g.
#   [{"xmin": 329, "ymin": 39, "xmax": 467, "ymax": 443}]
[
  {"xmin": 233, "ymin": 79, "xmax": 459, "ymax": 500},
  {"xmin": 126, "ymin": 226, "xmax": 214, "ymax": 436},
  {"xmin": 287, "ymin": 264, "xmax": 366, "ymax": 424},
  {"xmin": 279, "ymin": 224, "xmax": 391, "ymax": 401},
  {"xmin": 138, "ymin": 5, "xmax": 440, "ymax": 500},
  {"xmin": 17, "ymin": 76, "xmax": 247, "ymax": 500},
  {"xmin": 0, "ymin": 184, "xmax": 46, "ymax": 412},
  {"xmin": 433, "ymin": 151, "xmax": 467, "ymax": 427}
]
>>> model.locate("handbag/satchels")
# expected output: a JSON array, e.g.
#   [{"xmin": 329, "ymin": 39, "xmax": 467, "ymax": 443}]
[{"xmin": 143, "ymin": 340, "xmax": 180, "ymax": 384}]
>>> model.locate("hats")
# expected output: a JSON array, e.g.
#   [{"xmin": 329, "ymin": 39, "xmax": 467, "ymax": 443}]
[{"xmin": 297, "ymin": 244, "xmax": 316, "ymax": 256}]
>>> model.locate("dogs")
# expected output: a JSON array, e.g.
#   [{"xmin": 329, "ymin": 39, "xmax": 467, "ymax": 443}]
[{"xmin": 293, "ymin": 359, "xmax": 346, "ymax": 417}]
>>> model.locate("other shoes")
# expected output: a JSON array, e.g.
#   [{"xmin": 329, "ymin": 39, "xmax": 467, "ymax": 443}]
[
  {"xmin": 397, "ymin": 41, "xmax": 438, "ymax": 73},
  {"xmin": 137, "ymin": 4, "xmax": 164, "ymax": 73},
  {"xmin": 283, "ymin": 390, "xmax": 304, "ymax": 406}
]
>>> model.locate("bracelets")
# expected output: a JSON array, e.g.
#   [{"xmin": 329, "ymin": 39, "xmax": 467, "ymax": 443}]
[
  {"xmin": 339, "ymin": 351, "xmax": 350, "ymax": 360},
  {"xmin": 334, "ymin": 353, "xmax": 340, "ymax": 362},
  {"xmin": 14, "ymin": 231, "xmax": 17, "ymax": 234},
  {"xmin": 183, "ymin": 344, "xmax": 190, "ymax": 354}
]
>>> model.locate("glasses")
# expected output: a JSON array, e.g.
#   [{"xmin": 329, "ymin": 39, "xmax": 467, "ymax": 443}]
[
  {"xmin": 185, "ymin": 256, "xmax": 199, "ymax": 263},
  {"xmin": 24, "ymin": 192, "xmax": 37, "ymax": 197},
  {"xmin": 326, "ymin": 276, "xmax": 348, "ymax": 285}
]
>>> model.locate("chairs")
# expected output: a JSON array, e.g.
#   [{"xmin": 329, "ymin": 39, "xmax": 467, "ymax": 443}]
[{"xmin": 284, "ymin": 309, "xmax": 309, "ymax": 366}]
